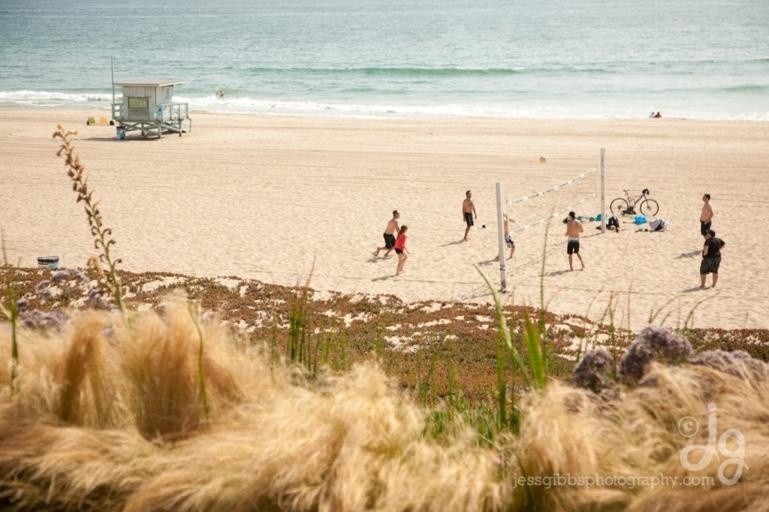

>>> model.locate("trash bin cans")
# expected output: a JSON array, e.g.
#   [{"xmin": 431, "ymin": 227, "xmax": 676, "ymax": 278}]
[
  {"xmin": 117, "ymin": 126, "xmax": 125, "ymax": 139},
  {"xmin": 38, "ymin": 256, "xmax": 59, "ymax": 269}
]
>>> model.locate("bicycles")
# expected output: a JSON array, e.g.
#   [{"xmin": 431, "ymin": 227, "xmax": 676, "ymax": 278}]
[{"xmin": 609, "ymin": 188, "xmax": 661, "ymax": 217}]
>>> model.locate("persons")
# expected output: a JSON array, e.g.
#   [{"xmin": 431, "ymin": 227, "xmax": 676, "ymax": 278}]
[
  {"xmin": 373, "ymin": 209, "xmax": 401, "ymax": 258},
  {"xmin": 565, "ymin": 212, "xmax": 585, "ymax": 272},
  {"xmin": 606, "ymin": 214, "xmax": 619, "ymax": 233},
  {"xmin": 700, "ymin": 193, "xmax": 713, "ymax": 241},
  {"xmin": 495, "ymin": 212, "xmax": 516, "ymax": 261},
  {"xmin": 649, "ymin": 112, "xmax": 654, "ymax": 118},
  {"xmin": 700, "ymin": 230, "xmax": 726, "ymax": 288},
  {"xmin": 655, "ymin": 111, "xmax": 663, "ymax": 118},
  {"xmin": 462, "ymin": 190, "xmax": 477, "ymax": 241},
  {"xmin": 394, "ymin": 225, "xmax": 410, "ymax": 276}
]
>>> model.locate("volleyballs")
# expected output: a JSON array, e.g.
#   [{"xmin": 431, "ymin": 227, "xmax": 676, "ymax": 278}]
[{"xmin": 540, "ymin": 157, "xmax": 545, "ymax": 163}]
